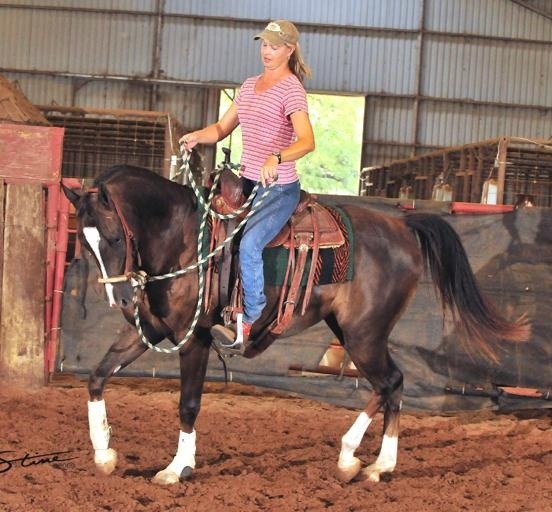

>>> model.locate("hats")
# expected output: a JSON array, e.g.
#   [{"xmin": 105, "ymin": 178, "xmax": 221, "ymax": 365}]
[{"xmin": 253, "ymin": 19, "xmax": 300, "ymax": 47}]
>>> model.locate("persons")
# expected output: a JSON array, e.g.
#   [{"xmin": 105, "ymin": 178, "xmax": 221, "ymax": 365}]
[{"xmin": 178, "ymin": 21, "xmax": 315, "ymax": 355}]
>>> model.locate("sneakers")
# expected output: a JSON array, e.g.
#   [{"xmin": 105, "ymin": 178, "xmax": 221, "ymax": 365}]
[{"xmin": 209, "ymin": 320, "xmax": 250, "ymax": 356}]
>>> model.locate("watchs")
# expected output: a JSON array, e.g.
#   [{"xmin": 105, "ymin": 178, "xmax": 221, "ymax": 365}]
[{"xmin": 272, "ymin": 150, "xmax": 281, "ymax": 165}]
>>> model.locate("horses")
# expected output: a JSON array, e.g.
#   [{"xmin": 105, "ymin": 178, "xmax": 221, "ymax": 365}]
[{"xmin": 56, "ymin": 163, "xmax": 532, "ymax": 488}]
